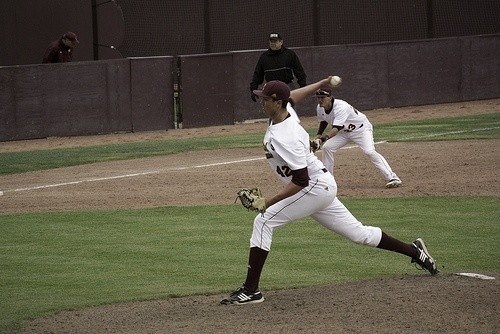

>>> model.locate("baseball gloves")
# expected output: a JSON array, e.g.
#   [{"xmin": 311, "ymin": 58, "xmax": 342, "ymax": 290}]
[
  {"xmin": 309, "ymin": 138, "xmax": 321, "ymax": 152},
  {"xmin": 236, "ymin": 187, "xmax": 267, "ymax": 214}
]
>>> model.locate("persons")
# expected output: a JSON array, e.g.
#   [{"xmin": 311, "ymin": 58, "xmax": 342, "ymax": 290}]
[
  {"xmin": 220, "ymin": 76, "xmax": 438, "ymax": 306},
  {"xmin": 250, "ymin": 33, "xmax": 307, "ymax": 103},
  {"xmin": 310, "ymin": 88, "xmax": 403, "ymax": 189},
  {"xmin": 42, "ymin": 32, "xmax": 80, "ymax": 63}
]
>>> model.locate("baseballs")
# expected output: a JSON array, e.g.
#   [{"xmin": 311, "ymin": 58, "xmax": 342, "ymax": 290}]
[{"xmin": 330, "ymin": 76, "xmax": 342, "ymax": 85}]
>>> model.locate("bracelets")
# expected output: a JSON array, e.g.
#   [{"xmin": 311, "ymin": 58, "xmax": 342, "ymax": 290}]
[{"xmin": 322, "ymin": 134, "xmax": 330, "ymax": 141}]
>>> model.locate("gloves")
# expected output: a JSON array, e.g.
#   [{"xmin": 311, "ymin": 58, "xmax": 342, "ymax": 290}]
[{"xmin": 251, "ymin": 89, "xmax": 260, "ymax": 102}]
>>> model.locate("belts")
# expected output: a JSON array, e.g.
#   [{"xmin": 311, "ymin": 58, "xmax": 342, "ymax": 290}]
[
  {"xmin": 359, "ymin": 123, "xmax": 363, "ymax": 128},
  {"xmin": 308, "ymin": 168, "xmax": 327, "ymax": 180}
]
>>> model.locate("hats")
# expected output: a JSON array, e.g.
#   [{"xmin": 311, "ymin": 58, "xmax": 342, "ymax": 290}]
[
  {"xmin": 269, "ymin": 32, "xmax": 282, "ymax": 41},
  {"xmin": 64, "ymin": 31, "xmax": 79, "ymax": 45},
  {"xmin": 252, "ymin": 80, "xmax": 291, "ymax": 100},
  {"xmin": 312, "ymin": 88, "xmax": 332, "ymax": 98}
]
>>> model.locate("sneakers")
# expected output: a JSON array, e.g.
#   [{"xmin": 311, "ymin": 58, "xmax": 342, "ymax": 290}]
[
  {"xmin": 385, "ymin": 179, "xmax": 403, "ymax": 188},
  {"xmin": 220, "ymin": 285, "xmax": 264, "ymax": 305},
  {"xmin": 411, "ymin": 238, "xmax": 438, "ymax": 275}
]
